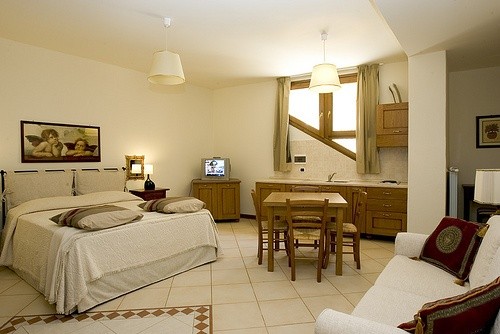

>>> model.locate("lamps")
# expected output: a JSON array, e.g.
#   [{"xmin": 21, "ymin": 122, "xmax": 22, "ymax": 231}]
[
  {"xmin": 146, "ymin": 18, "xmax": 185, "ymax": 86},
  {"xmin": 132, "ymin": 163, "xmax": 141, "ymax": 176},
  {"xmin": 144, "ymin": 164, "xmax": 155, "ymax": 190},
  {"xmin": 309, "ymin": 30, "xmax": 342, "ymax": 94}
]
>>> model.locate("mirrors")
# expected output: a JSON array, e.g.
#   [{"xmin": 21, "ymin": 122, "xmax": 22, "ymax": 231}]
[{"xmin": 126, "ymin": 155, "xmax": 144, "ymax": 180}]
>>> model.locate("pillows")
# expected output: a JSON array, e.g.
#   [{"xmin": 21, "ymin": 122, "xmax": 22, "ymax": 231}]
[
  {"xmin": 48, "ymin": 205, "xmax": 144, "ymax": 230},
  {"xmin": 398, "ymin": 276, "xmax": 500, "ymax": 334},
  {"xmin": 408, "ymin": 215, "xmax": 490, "ymax": 285},
  {"xmin": 137, "ymin": 197, "xmax": 206, "ymax": 213}
]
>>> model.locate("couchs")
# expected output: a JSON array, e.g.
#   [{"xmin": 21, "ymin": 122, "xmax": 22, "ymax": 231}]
[{"xmin": 313, "ymin": 215, "xmax": 500, "ymax": 334}]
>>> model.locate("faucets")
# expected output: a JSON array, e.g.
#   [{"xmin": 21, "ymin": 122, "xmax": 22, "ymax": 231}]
[{"xmin": 324, "ymin": 172, "xmax": 336, "ymax": 182}]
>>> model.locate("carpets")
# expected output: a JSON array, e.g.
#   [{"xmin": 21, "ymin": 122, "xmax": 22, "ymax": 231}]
[{"xmin": 0, "ymin": 305, "xmax": 213, "ymax": 334}]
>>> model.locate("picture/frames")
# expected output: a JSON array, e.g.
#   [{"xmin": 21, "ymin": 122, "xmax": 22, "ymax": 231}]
[
  {"xmin": 476, "ymin": 115, "xmax": 500, "ymax": 149},
  {"xmin": 21, "ymin": 120, "xmax": 101, "ymax": 163}
]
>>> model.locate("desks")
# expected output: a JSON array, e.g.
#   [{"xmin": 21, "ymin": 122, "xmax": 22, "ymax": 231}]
[{"xmin": 263, "ymin": 192, "xmax": 349, "ymax": 274}]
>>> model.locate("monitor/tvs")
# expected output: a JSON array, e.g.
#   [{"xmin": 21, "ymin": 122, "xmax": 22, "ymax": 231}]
[{"xmin": 201, "ymin": 158, "xmax": 230, "ymax": 181}]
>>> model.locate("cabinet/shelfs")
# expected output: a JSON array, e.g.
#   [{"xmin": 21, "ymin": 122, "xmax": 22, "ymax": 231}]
[
  {"xmin": 193, "ymin": 178, "xmax": 241, "ymax": 222},
  {"xmin": 255, "ymin": 179, "xmax": 407, "ymax": 237},
  {"xmin": 375, "ymin": 101, "xmax": 408, "ymax": 147}
]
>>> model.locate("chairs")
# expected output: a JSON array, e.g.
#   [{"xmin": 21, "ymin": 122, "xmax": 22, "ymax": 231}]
[{"xmin": 251, "ymin": 189, "xmax": 367, "ymax": 283}]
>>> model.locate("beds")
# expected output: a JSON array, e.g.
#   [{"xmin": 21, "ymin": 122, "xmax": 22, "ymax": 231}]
[{"xmin": 0, "ymin": 167, "xmax": 224, "ymax": 315}]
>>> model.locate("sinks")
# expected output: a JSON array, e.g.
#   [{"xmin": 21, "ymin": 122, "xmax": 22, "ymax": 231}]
[{"xmin": 333, "ymin": 181, "xmax": 350, "ymax": 183}]
